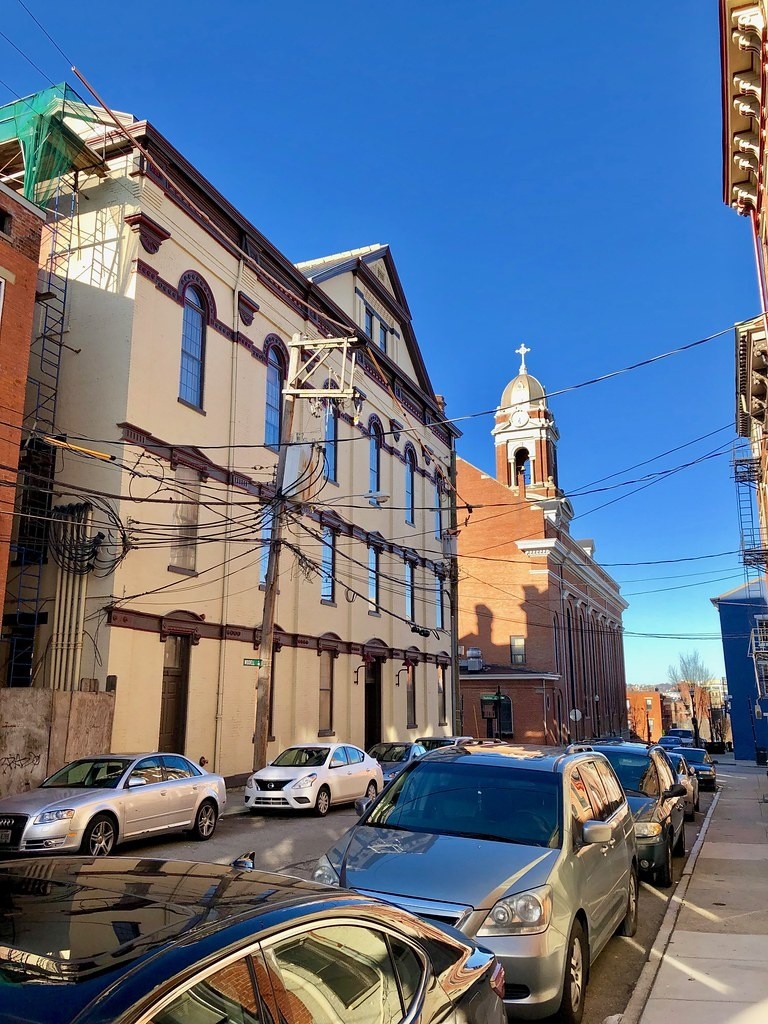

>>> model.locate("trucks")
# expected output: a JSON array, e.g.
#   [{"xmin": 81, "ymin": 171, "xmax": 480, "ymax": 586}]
[{"xmin": 669, "ymin": 728, "xmax": 706, "ymax": 749}]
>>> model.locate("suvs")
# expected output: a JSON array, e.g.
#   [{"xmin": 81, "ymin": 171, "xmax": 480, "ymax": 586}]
[
  {"xmin": 552, "ymin": 738, "xmax": 688, "ymax": 889},
  {"xmin": 311, "ymin": 738, "xmax": 639, "ymax": 1024}
]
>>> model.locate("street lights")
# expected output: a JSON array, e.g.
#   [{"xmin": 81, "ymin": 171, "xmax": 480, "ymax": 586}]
[{"xmin": 251, "ymin": 492, "xmax": 394, "ymax": 776}]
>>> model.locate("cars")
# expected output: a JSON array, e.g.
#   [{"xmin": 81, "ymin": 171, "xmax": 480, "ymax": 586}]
[
  {"xmin": 415, "ymin": 735, "xmax": 474, "ymax": 753},
  {"xmin": 672, "ymin": 747, "xmax": 719, "ymax": 792},
  {"xmin": 0, "ymin": 752, "xmax": 228, "ymax": 858},
  {"xmin": 364, "ymin": 741, "xmax": 430, "ymax": 790},
  {"xmin": 657, "ymin": 752, "xmax": 700, "ymax": 821},
  {"xmin": 245, "ymin": 743, "xmax": 384, "ymax": 819},
  {"xmin": 656, "ymin": 736, "xmax": 683, "ymax": 749},
  {"xmin": 1, "ymin": 854, "xmax": 508, "ymax": 1024}
]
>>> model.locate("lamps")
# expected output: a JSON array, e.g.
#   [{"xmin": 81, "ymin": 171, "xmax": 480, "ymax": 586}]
[
  {"xmin": 361, "ymin": 653, "xmax": 376, "ymax": 671},
  {"xmin": 401, "ymin": 657, "xmax": 415, "ymax": 674}
]
